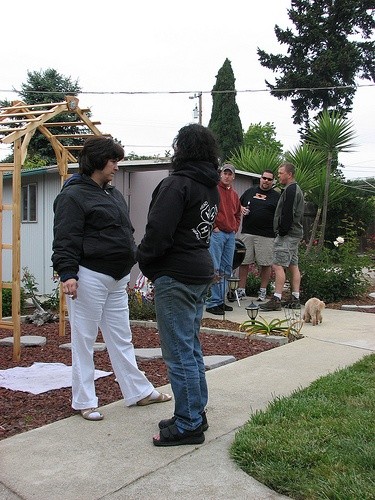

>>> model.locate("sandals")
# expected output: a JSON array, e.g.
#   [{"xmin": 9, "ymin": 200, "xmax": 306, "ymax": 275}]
[
  {"xmin": 159, "ymin": 413, "xmax": 209, "ymax": 431},
  {"xmin": 81, "ymin": 408, "xmax": 104, "ymax": 420},
  {"xmin": 153, "ymin": 424, "xmax": 205, "ymax": 446},
  {"xmin": 138, "ymin": 392, "xmax": 172, "ymax": 406}
]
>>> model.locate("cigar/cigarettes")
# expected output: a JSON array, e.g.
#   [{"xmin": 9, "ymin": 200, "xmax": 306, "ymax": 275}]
[{"xmin": 67, "ymin": 295, "xmax": 74, "ymax": 298}]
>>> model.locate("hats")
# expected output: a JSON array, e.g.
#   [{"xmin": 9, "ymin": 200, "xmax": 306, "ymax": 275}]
[{"xmin": 222, "ymin": 164, "xmax": 235, "ymax": 174}]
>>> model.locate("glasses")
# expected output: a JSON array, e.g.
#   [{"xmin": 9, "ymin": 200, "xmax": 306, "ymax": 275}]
[{"xmin": 262, "ymin": 177, "xmax": 273, "ymax": 181}]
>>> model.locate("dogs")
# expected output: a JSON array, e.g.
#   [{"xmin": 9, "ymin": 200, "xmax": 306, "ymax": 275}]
[{"xmin": 302, "ymin": 298, "xmax": 325, "ymax": 326}]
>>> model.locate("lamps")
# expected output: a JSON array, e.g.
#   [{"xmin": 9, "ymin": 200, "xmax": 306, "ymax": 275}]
[
  {"xmin": 226, "ymin": 273, "xmax": 241, "ymax": 291},
  {"xmin": 245, "ymin": 300, "xmax": 260, "ymax": 319}
]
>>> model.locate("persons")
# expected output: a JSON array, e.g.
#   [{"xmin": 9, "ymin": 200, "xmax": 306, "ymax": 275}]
[
  {"xmin": 51, "ymin": 136, "xmax": 172, "ymax": 421},
  {"xmin": 136, "ymin": 124, "xmax": 219, "ymax": 446},
  {"xmin": 229, "ymin": 170, "xmax": 281, "ymax": 302},
  {"xmin": 206, "ymin": 164, "xmax": 241, "ymax": 315},
  {"xmin": 257, "ymin": 163, "xmax": 304, "ymax": 311}
]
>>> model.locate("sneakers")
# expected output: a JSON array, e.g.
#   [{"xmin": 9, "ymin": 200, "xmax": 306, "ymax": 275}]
[
  {"xmin": 259, "ymin": 296, "xmax": 282, "ymax": 311},
  {"xmin": 218, "ymin": 303, "xmax": 233, "ymax": 311},
  {"xmin": 281, "ymin": 295, "xmax": 301, "ymax": 309},
  {"xmin": 206, "ymin": 306, "xmax": 224, "ymax": 315}
]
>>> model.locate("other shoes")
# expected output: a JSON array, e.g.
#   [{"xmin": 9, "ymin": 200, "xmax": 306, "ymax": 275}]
[
  {"xmin": 257, "ymin": 291, "xmax": 266, "ymax": 301},
  {"xmin": 238, "ymin": 290, "xmax": 246, "ymax": 301}
]
